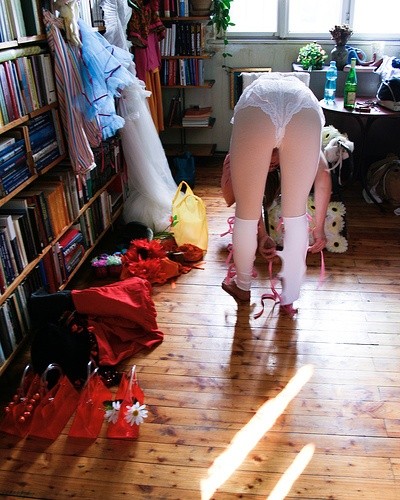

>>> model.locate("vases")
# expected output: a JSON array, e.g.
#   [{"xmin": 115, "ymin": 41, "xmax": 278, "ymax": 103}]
[{"xmin": 331, "ymin": 45, "xmax": 348, "ymax": 71}]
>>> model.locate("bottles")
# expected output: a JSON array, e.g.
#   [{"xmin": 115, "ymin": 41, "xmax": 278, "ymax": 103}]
[
  {"xmin": 324, "ymin": 61, "xmax": 337, "ymax": 100},
  {"xmin": 344, "ymin": 57, "xmax": 358, "ymax": 109}
]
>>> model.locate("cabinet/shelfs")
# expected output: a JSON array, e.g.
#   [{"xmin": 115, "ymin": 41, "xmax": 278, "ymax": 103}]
[
  {"xmin": 160, "ymin": 16, "xmax": 218, "ymax": 159},
  {"xmin": 0, "ymin": 0, "xmax": 128, "ymax": 376}
]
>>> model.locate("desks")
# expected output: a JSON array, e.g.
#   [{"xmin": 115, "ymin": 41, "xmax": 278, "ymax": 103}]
[{"xmin": 320, "ymin": 96, "xmax": 400, "ymax": 187}]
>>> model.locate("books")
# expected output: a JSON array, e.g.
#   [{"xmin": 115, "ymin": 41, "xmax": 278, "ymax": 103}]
[{"xmin": 0, "ymin": 0, "xmax": 213, "ymax": 366}]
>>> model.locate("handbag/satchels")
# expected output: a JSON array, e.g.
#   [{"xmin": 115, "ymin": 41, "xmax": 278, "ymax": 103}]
[
  {"xmin": 28, "ymin": 363, "xmax": 80, "ymax": 440},
  {"xmin": 170, "ymin": 181, "xmax": 209, "ymax": 252},
  {"xmin": 376, "ymin": 76, "xmax": 400, "ymax": 112},
  {"xmin": 106, "ymin": 365, "xmax": 145, "ymax": 441},
  {"xmin": 173, "ymin": 152, "xmax": 196, "ymax": 188},
  {"xmin": 0, "ymin": 365, "xmax": 48, "ymax": 436},
  {"xmin": 66, "ymin": 361, "xmax": 111, "ymax": 438}
]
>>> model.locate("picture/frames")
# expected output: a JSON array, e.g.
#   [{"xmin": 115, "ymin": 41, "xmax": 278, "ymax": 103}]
[{"xmin": 230, "ymin": 68, "xmax": 271, "ymax": 109}]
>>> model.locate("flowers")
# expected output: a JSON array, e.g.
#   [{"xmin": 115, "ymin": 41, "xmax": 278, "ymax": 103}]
[{"xmin": 330, "ymin": 25, "xmax": 353, "ymax": 46}]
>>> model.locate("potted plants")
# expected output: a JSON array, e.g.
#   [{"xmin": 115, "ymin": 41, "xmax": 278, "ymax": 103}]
[
  {"xmin": 297, "ymin": 43, "xmax": 328, "ymax": 70},
  {"xmin": 190, "ymin": 0, "xmax": 236, "ymax": 76}
]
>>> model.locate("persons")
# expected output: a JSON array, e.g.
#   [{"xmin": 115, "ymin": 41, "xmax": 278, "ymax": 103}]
[{"xmin": 222, "ymin": 74, "xmax": 331, "ymax": 319}]
